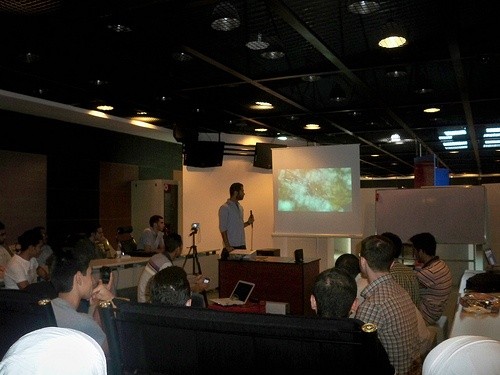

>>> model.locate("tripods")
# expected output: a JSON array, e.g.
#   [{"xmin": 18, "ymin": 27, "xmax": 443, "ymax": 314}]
[{"xmin": 182, "ymin": 228, "xmax": 207, "ymax": 302}]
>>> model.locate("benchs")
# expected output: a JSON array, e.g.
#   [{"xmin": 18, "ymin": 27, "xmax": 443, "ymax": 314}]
[{"xmin": 0, "ymin": 284, "xmax": 395, "ymax": 375}]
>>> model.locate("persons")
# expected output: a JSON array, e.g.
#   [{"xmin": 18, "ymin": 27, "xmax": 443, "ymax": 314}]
[
  {"xmin": 381, "ymin": 231, "xmax": 420, "ymax": 309},
  {"xmin": 3, "ymin": 228, "xmax": 44, "ymax": 291},
  {"xmin": 87, "ymin": 225, "xmax": 117, "ymax": 259},
  {"xmin": 137, "ymin": 232, "xmax": 212, "ymax": 311},
  {"xmin": 0, "ymin": 221, "xmax": 13, "ymax": 279},
  {"xmin": 33, "ymin": 225, "xmax": 53, "ymax": 265},
  {"xmin": 46, "ymin": 253, "xmax": 113, "ymax": 358},
  {"xmin": 350, "ymin": 234, "xmax": 419, "ymax": 375},
  {"xmin": 149, "ymin": 265, "xmax": 192, "ymax": 307},
  {"xmin": 310, "ymin": 267, "xmax": 397, "ymax": 375},
  {"xmin": 410, "ymin": 232, "xmax": 453, "ymax": 326},
  {"xmin": 335, "ymin": 255, "xmax": 368, "ymax": 303},
  {"xmin": 137, "ymin": 215, "xmax": 166, "ymax": 254},
  {"xmin": 218, "ymin": 183, "xmax": 254, "ymax": 259}
]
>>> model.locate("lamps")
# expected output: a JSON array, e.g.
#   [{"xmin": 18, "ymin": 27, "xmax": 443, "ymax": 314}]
[{"xmin": 96, "ymin": 0, "xmax": 444, "ymax": 146}]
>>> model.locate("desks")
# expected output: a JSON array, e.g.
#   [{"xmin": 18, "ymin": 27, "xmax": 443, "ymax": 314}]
[
  {"xmin": 217, "ymin": 259, "xmax": 322, "ymax": 314},
  {"xmin": 272, "ymin": 233, "xmax": 362, "ymax": 271},
  {"xmin": 90, "ymin": 248, "xmax": 220, "ymax": 293},
  {"xmin": 450, "ymin": 270, "xmax": 500, "ymax": 341}
]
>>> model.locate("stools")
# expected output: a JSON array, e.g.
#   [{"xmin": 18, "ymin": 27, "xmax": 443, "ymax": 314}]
[{"xmin": 427, "ymin": 316, "xmax": 448, "ymax": 346}]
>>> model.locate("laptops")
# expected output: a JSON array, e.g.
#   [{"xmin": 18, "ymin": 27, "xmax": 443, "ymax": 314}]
[
  {"xmin": 209, "ymin": 280, "xmax": 255, "ymax": 306},
  {"xmin": 483, "ymin": 248, "xmax": 500, "ymax": 266}
]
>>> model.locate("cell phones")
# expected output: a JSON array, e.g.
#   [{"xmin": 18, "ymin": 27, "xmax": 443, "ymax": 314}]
[
  {"xmin": 203, "ymin": 278, "xmax": 210, "ymax": 284},
  {"xmin": 100, "ymin": 266, "xmax": 111, "ymax": 284}
]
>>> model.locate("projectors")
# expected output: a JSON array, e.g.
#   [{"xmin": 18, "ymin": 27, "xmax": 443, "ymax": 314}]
[{"xmin": 229, "ymin": 249, "xmax": 257, "ymax": 259}]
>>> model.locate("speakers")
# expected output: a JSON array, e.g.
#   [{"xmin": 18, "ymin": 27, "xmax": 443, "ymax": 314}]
[
  {"xmin": 183, "ymin": 140, "xmax": 224, "ymax": 168},
  {"xmin": 295, "ymin": 248, "xmax": 303, "ymax": 263},
  {"xmin": 253, "ymin": 143, "xmax": 287, "ymax": 169}
]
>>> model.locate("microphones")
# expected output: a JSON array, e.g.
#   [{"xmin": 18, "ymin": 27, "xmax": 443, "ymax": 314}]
[{"xmin": 250, "ymin": 210, "xmax": 254, "ymax": 228}]
